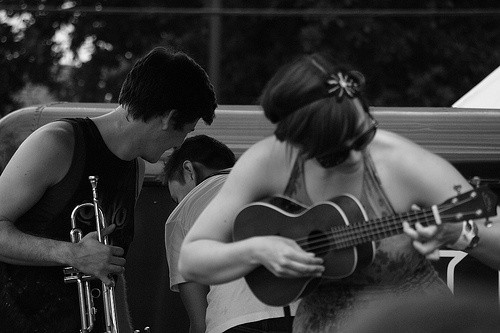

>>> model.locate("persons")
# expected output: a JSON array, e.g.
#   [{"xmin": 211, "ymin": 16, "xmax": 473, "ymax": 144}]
[
  {"xmin": 0, "ymin": 46, "xmax": 219, "ymax": 333},
  {"xmin": 177, "ymin": 50, "xmax": 500, "ymax": 333},
  {"xmin": 162, "ymin": 134, "xmax": 301, "ymax": 333}
]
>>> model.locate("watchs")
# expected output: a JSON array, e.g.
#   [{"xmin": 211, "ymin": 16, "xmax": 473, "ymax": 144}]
[{"xmin": 462, "ymin": 223, "xmax": 481, "ymax": 252}]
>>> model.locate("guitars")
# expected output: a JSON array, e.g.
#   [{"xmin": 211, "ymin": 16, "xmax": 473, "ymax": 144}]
[{"xmin": 233, "ymin": 173, "xmax": 497, "ymax": 307}]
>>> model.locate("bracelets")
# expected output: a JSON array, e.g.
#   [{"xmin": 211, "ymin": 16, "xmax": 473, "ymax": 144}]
[{"xmin": 446, "ymin": 219, "xmax": 474, "ymax": 250}]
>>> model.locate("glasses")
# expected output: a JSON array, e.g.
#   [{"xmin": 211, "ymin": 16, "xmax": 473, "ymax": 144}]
[{"xmin": 316, "ymin": 109, "xmax": 378, "ymax": 169}]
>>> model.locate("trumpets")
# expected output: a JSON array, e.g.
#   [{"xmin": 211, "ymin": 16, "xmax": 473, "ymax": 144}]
[{"xmin": 62, "ymin": 176, "xmax": 122, "ymax": 333}]
